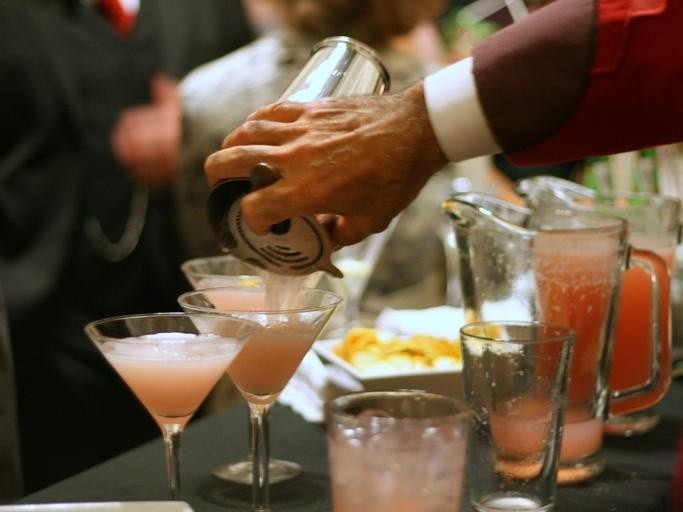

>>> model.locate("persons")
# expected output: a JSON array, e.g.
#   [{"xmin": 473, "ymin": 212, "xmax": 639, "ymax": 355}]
[
  {"xmin": 200, "ymin": 0, "xmax": 683, "ymax": 250},
  {"xmin": 170, "ymin": 2, "xmax": 530, "ymax": 416},
  {"xmin": 435, "ymin": 0, "xmax": 683, "ymax": 211},
  {"xmin": 0, "ymin": 2, "xmax": 209, "ymax": 502}
]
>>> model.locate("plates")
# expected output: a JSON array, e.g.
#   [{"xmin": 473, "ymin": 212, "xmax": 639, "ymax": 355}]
[{"xmin": 315, "ymin": 328, "xmax": 469, "ymax": 387}]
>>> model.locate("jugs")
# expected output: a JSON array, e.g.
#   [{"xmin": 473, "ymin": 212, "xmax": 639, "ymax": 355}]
[{"xmin": 438, "ymin": 189, "xmax": 683, "ymax": 482}]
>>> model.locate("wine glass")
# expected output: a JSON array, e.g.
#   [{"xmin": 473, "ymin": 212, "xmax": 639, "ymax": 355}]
[
  {"xmin": 87, "ymin": 311, "xmax": 255, "ymax": 502},
  {"xmin": 178, "ymin": 287, "xmax": 342, "ymax": 510},
  {"xmin": 183, "ymin": 255, "xmax": 332, "ymax": 485}
]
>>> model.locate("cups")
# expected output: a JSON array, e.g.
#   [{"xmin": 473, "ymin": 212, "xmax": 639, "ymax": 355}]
[
  {"xmin": 458, "ymin": 320, "xmax": 576, "ymax": 511},
  {"xmin": 331, "ymin": 393, "xmax": 472, "ymax": 509},
  {"xmin": 206, "ymin": 36, "xmax": 392, "ymax": 276}
]
